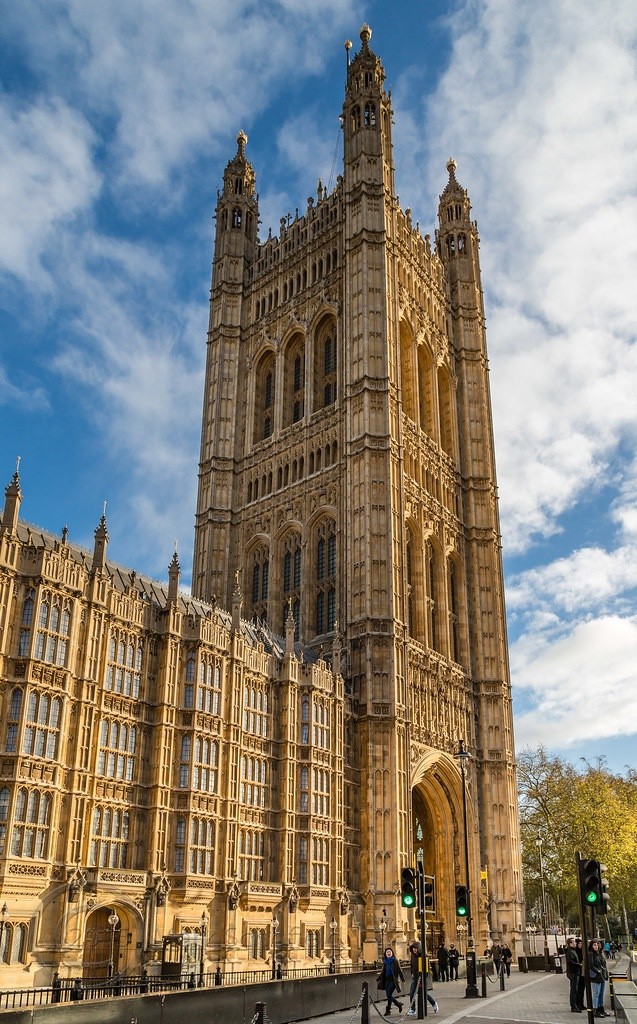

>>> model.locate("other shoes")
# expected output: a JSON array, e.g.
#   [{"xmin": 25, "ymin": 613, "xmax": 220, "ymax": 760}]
[
  {"xmin": 384, "ymin": 1006, "xmax": 391, "ymax": 1016},
  {"xmin": 394, "ymin": 1000, "xmax": 403, "ymax": 1013},
  {"xmin": 432, "ymin": 1002, "xmax": 438, "ymax": 1013},
  {"xmin": 407, "ymin": 1010, "xmax": 416, "ymax": 1016},
  {"xmin": 593, "ymin": 1008, "xmax": 605, "ymax": 1018},
  {"xmin": 599, "ymin": 1006, "xmax": 609, "ymax": 1017}
]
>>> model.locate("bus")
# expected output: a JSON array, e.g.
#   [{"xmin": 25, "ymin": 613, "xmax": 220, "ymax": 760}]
[{"xmin": 416, "ymin": 918, "xmax": 446, "ymax": 959}]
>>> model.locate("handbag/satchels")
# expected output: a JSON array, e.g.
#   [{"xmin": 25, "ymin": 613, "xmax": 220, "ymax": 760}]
[
  {"xmin": 377, "ymin": 974, "xmax": 386, "ymax": 990},
  {"xmin": 507, "ymin": 957, "xmax": 511, "ymax": 962}
]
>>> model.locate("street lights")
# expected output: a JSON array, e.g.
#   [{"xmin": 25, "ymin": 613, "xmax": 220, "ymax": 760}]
[
  {"xmin": 198, "ymin": 911, "xmax": 209, "ymax": 987},
  {"xmin": 379, "ymin": 918, "xmax": 387, "ymax": 960},
  {"xmin": 526, "ymin": 924, "xmax": 537, "ymax": 956},
  {"xmin": 271, "ymin": 914, "xmax": 281, "ymax": 981},
  {"xmin": 453, "ymin": 739, "xmax": 482, "ymax": 999},
  {"xmin": 108, "ymin": 906, "xmax": 120, "ymax": 978},
  {"xmin": 535, "ymin": 835, "xmax": 551, "ymax": 972},
  {"xmin": 329, "ymin": 917, "xmax": 339, "ymax": 974},
  {"xmin": 456, "ymin": 921, "xmax": 469, "ymax": 958}
]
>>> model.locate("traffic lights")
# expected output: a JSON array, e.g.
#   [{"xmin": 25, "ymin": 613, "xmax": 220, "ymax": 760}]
[
  {"xmin": 401, "ymin": 867, "xmax": 416, "ymax": 908},
  {"xmin": 422, "ymin": 883, "xmax": 432, "ymax": 906},
  {"xmin": 455, "ymin": 886, "xmax": 467, "ymax": 916},
  {"xmin": 581, "ymin": 860, "xmax": 603, "ymax": 905},
  {"xmin": 598, "ymin": 863, "xmax": 610, "ymax": 902}
]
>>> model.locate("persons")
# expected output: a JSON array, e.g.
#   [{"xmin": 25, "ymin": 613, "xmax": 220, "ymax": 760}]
[
  {"xmin": 484, "ymin": 941, "xmax": 512, "ymax": 978},
  {"xmin": 565, "ymin": 937, "xmax": 611, "ymax": 1018},
  {"xmin": 604, "ymin": 941, "xmax": 622, "ymax": 960},
  {"xmin": 558, "ymin": 945, "xmax": 566, "ymax": 955},
  {"xmin": 406, "ymin": 942, "xmax": 439, "ymax": 1015},
  {"xmin": 437, "ymin": 942, "xmax": 460, "ymax": 982},
  {"xmin": 376, "ymin": 948, "xmax": 406, "ymax": 1016}
]
